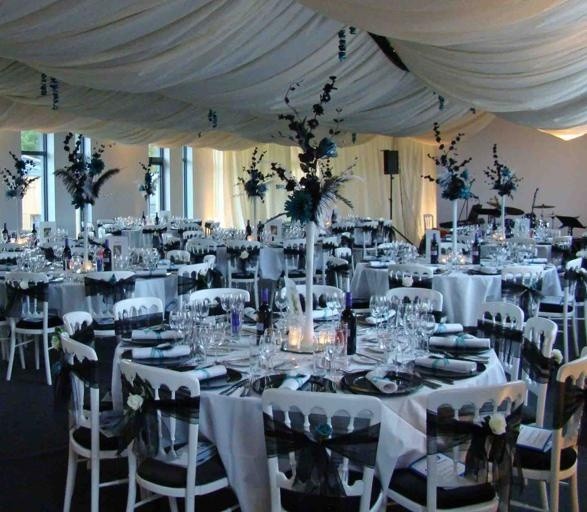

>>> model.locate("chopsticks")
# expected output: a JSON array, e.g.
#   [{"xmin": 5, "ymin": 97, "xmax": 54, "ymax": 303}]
[
  {"xmin": 423, "ymin": 380, "xmax": 441, "ymax": 389},
  {"xmin": 219, "ymin": 379, "xmax": 249, "ymax": 396}
]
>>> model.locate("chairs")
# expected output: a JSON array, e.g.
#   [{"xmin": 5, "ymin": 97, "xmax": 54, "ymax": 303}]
[
  {"xmin": 538, "ymin": 258, "xmax": 581, "ymax": 364},
  {"xmin": 476, "ymin": 302, "xmax": 525, "ymax": 383},
  {"xmin": 517, "ymin": 318, "xmax": 559, "ymax": 429},
  {"xmin": 277, "ymin": 286, "xmax": 347, "ymax": 313},
  {"xmin": 499, "ymin": 266, "xmax": 545, "ymax": 322},
  {"xmin": 383, "ymin": 265, "xmax": 436, "ymax": 292},
  {"xmin": 385, "ymin": 382, "xmax": 531, "ymax": 510},
  {"xmin": 510, "ymin": 358, "xmax": 586, "ymax": 512},
  {"xmin": 60, "ymin": 332, "xmax": 129, "ymax": 512},
  {"xmin": 326, "ymin": 256, "xmax": 371, "ymax": 307},
  {"xmin": 187, "ymin": 289, "xmax": 251, "ymax": 317},
  {"xmin": 263, "ymin": 389, "xmax": 386, "ymax": 511},
  {"xmin": 118, "ymin": 358, "xmax": 244, "ymax": 512},
  {"xmin": 63, "ymin": 312, "xmax": 126, "ymax": 430},
  {"xmin": 85, "ymin": 270, "xmax": 138, "ymax": 346},
  {"xmin": 163, "ymin": 264, "xmax": 213, "ymax": 318},
  {"xmin": 167, "ymin": 251, "xmax": 191, "ymax": 265},
  {"xmin": 3, "ymin": 273, "xmax": 64, "ymax": 387},
  {"xmin": 384, "ymin": 288, "xmax": 445, "ymax": 323},
  {"xmin": 203, "ymin": 256, "xmax": 216, "ymax": 274},
  {"xmin": 112, "ymin": 299, "xmax": 165, "ymax": 345}
]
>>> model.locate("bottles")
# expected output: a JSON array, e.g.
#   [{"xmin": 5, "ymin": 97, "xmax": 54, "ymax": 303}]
[
  {"xmin": 431, "ymin": 234, "xmax": 439, "ymax": 263},
  {"xmin": 257, "ymin": 222, "xmax": 264, "ymax": 241},
  {"xmin": 32, "ymin": 224, "xmax": 38, "ymax": 246},
  {"xmin": 103, "ymin": 239, "xmax": 112, "ymax": 271},
  {"xmin": 62, "ymin": 238, "xmax": 72, "ymax": 271},
  {"xmin": 2, "ymin": 223, "xmax": 8, "ymax": 244},
  {"xmin": 473, "ymin": 234, "xmax": 481, "ymax": 264},
  {"xmin": 256, "ymin": 287, "xmax": 273, "ymax": 345},
  {"xmin": 340, "ymin": 292, "xmax": 356, "ymax": 355},
  {"xmin": 246, "ymin": 220, "xmax": 251, "ymax": 239},
  {"xmin": 331, "ymin": 209, "xmax": 336, "ymax": 223}
]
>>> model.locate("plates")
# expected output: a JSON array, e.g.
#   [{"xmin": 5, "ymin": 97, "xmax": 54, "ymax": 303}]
[
  {"xmin": 121, "ymin": 331, "xmax": 183, "ymax": 346},
  {"xmin": 342, "ymin": 371, "xmax": 423, "ymax": 397},
  {"xmin": 365, "ymin": 310, "xmax": 447, "ymax": 329},
  {"xmin": 478, "ymin": 270, "xmax": 501, "ymax": 275},
  {"xmin": 424, "ymin": 272, "xmax": 441, "ymax": 274},
  {"xmin": 122, "ymin": 349, "xmax": 195, "ymax": 366},
  {"xmin": 405, "ymin": 358, "xmax": 485, "ymax": 379},
  {"xmin": 243, "ymin": 313, "xmax": 281, "ymax": 324},
  {"xmin": 363, "ymin": 259, "xmax": 389, "ymax": 262},
  {"xmin": 428, "ymin": 347, "xmax": 491, "ymax": 355},
  {"xmin": 367, "ymin": 265, "xmax": 388, "ymax": 269},
  {"xmin": 252, "ymin": 374, "xmax": 336, "ymax": 395},
  {"xmin": 432, "ymin": 332, "xmax": 464, "ymax": 337},
  {"xmin": 169, "ymin": 368, "xmax": 242, "ymax": 390},
  {"xmin": 313, "ymin": 314, "xmax": 341, "ymax": 322}
]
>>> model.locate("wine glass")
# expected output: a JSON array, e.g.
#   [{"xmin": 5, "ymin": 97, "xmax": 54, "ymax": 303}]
[
  {"xmin": 130, "ymin": 248, "xmax": 160, "ymax": 270},
  {"xmin": 16, "ymin": 247, "xmax": 63, "ymax": 280},
  {"xmin": 326, "ymin": 293, "xmax": 346, "ymax": 328},
  {"xmin": 221, "ymin": 294, "xmax": 244, "ymax": 325},
  {"xmin": 250, "ymin": 328, "xmax": 282, "ymax": 377},
  {"xmin": 113, "ymin": 259, "xmax": 129, "ymax": 270},
  {"xmin": 66, "ymin": 256, "xmax": 84, "ymax": 283},
  {"xmin": 275, "ymin": 292, "xmax": 288, "ymax": 319},
  {"xmin": 370, "ymin": 296, "xmax": 435, "ymax": 370},
  {"xmin": 315, "ymin": 330, "xmax": 348, "ymax": 376},
  {"xmin": 171, "ymin": 294, "xmax": 226, "ymax": 365}
]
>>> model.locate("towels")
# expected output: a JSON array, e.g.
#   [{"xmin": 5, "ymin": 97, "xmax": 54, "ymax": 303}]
[
  {"xmin": 524, "ymin": 263, "xmax": 546, "ymax": 270},
  {"xmin": 0, "ymin": 264, "xmax": 16, "ymax": 270},
  {"xmin": 311, "ymin": 308, "xmax": 339, "ymax": 319},
  {"xmin": 278, "ymin": 371, "xmax": 311, "ymax": 391},
  {"xmin": 365, "ymin": 309, "xmax": 396, "ymax": 324},
  {"xmin": 132, "ymin": 345, "xmax": 191, "ymax": 358},
  {"xmin": 435, "ymin": 323, "xmax": 463, "ymax": 333},
  {"xmin": 184, "ymin": 364, "xmax": 227, "ymax": 381},
  {"xmin": 524, "ymin": 258, "xmax": 546, "ymax": 263},
  {"xmin": 368, "ymin": 261, "xmax": 396, "ymax": 267},
  {"xmin": 473, "ymin": 264, "xmax": 497, "ymax": 275},
  {"xmin": 244, "ymin": 307, "xmax": 260, "ymax": 321},
  {"xmin": 365, "ymin": 367, "xmax": 398, "ymax": 394},
  {"xmin": 43, "ymin": 272, "xmax": 55, "ymax": 280},
  {"xmin": 159, "ymin": 259, "xmax": 171, "ymax": 264},
  {"xmin": 136, "ymin": 270, "xmax": 166, "ymax": 276},
  {"xmin": 429, "ymin": 336, "xmax": 490, "ymax": 348},
  {"xmin": 271, "ymin": 241, "xmax": 283, "ymax": 246},
  {"xmin": 415, "ymin": 357, "xmax": 478, "ymax": 374},
  {"xmin": 413, "ymin": 266, "xmax": 440, "ymax": 274},
  {"xmin": 144, "ymin": 264, "xmax": 170, "ymax": 269},
  {"xmin": 132, "ymin": 330, "xmax": 177, "ymax": 339},
  {"xmin": 178, "ymin": 309, "xmax": 216, "ymax": 325},
  {"xmin": 363, "ymin": 255, "xmax": 387, "ymax": 261}
]
